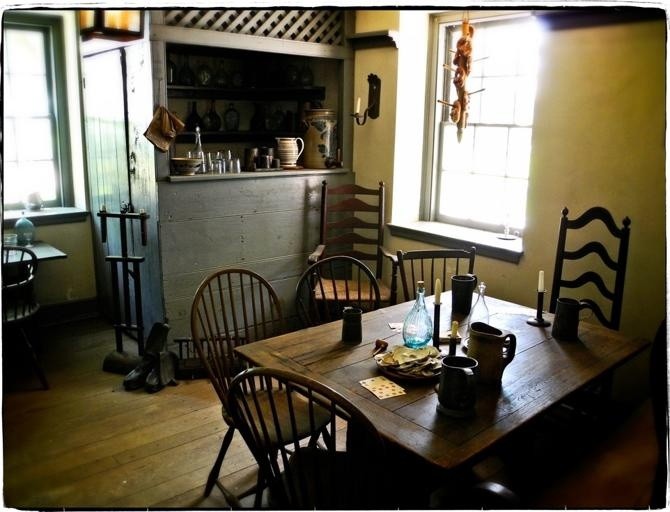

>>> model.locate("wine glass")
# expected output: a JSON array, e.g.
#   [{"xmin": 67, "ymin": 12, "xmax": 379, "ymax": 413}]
[{"xmin": 23, "ymin": 232, "xmax": 34, "ymax": 248}]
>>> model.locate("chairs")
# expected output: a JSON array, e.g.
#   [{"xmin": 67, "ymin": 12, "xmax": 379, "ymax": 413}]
[
  {"xmin": 295, "ymin": 255, "xmax": 381, "ymax": 325},
  {"xmin": 3, "ymin": 245, "xmax": 51, "ymax": 397},
  {"xmin": 226, "ymin": 368, "xmax": 386, "ymax": 509},
  {"xmin": 307, "ymin": 180, "xmax": 400, "ymax": 308},
  {"xmin": 395, "ymin": 245, "xmax": 479, "ymax": 298},
  {"xmin": 547, "ymin": 205, "xmax": 632, "ymax": 332},
  {"xmin": 190, "ymin": 266, "xmax": 339, "ymax": 507}
]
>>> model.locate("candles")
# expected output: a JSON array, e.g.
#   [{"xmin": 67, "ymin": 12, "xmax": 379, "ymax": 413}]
[
  {"xmin": 433, "ymin": 276, "xmax": 442, "ymax": 304},
  {"xmin": 450, "ymin": 321, "xmax": 459, "ymax": 339},
  {"xmin": 538, "ymin": 270, "xmax": 545, "ymax": 293},
  {"xmin": 355, "ymin": 96, "xmax": 363, "ymax": 114}
]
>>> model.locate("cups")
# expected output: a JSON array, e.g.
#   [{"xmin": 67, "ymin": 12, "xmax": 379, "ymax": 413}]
[
  {"xmin": 245, "ymin": 147, "xmax": 282, "ymax": 171},
  {"xmin": 553, "ymin": 296, "xmax": 593, "ymax": 341},
  {"xmin": 341, "ymin": 305, "xmax": 363, "ymax": 343},
  {"xmin": 468, "ymin": 323, "xmax": 518, "ymax": 384},
  {"xmin": 438, "ymin": 354, "xmax": 479, "ymax": 414},
  {"xmin": 204, "ymin": 152, "xmax": 240, "ymax": 174},
  {"xmin": 451, "ymin": 274, "xmax": 478, "ymax": 314},
  {"xmin": 3, "ymin": 233, "xmax": 17, "ymax": 255}
]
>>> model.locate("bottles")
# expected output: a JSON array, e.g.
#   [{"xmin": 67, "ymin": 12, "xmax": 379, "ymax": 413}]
[
  {"xmin": 14, "ymin": 211, "xmax": 34, "ymax": 243},
  {"xmin": 189, "ymin": 131, "xmax": 206, "ymax": 173},
  {"xmin": 185, "ymin": 99, "xmax": 240, "ymax": 132},
  {"xmin": 166, "ymin": 59, "xmax": 244, "ymax": 87},
  {"xmin": 465, "ymin": 283, "xmax": 490, "ymax": 342},
  {"xmin": 259, "ymin": 105, "xmax": 297, "ymax": 150},
  {"xmin": 402, "ymin": 281, "xmax": 433, "ymax": 346}
]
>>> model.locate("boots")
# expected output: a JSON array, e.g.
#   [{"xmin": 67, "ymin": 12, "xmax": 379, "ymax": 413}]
[
  {"xmin": 144, "ymin": 351, "xmax": 178, "ymax": 394},
  {"xmin": 123, "ymin": 321, "xmax": 170, "ymax": 391}
]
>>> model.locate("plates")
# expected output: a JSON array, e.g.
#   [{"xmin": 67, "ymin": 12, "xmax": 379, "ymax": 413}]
[{"xmin": 375, "ymin": 354, "xmax": 445, "ymax": 382}]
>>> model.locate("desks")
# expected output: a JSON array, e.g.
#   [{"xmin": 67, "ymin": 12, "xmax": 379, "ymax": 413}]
[{"xmin": 4, "ymin": 241, "xmax": 67, "ymax": 270}]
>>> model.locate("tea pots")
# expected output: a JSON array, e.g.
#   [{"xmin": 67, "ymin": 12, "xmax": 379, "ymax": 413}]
[{"xmin": 275, "ymin": 138, "xmax": 304, "ymax": 165}]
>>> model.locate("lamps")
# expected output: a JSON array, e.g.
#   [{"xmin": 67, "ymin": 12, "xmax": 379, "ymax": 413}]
[{"xmin": 77, "ymin": 8, "xmax": 145, "ymax": 42}]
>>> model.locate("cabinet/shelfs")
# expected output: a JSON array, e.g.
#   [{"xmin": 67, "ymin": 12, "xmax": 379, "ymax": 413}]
[{"xmin": 168, "ymin": 48, "xmax": 326, "ymax": 148}]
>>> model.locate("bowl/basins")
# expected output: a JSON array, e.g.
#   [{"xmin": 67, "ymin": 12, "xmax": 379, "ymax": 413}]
[{"xmin": 172, "ymin": 157, "xmax": 202, "ymax": 176}]
